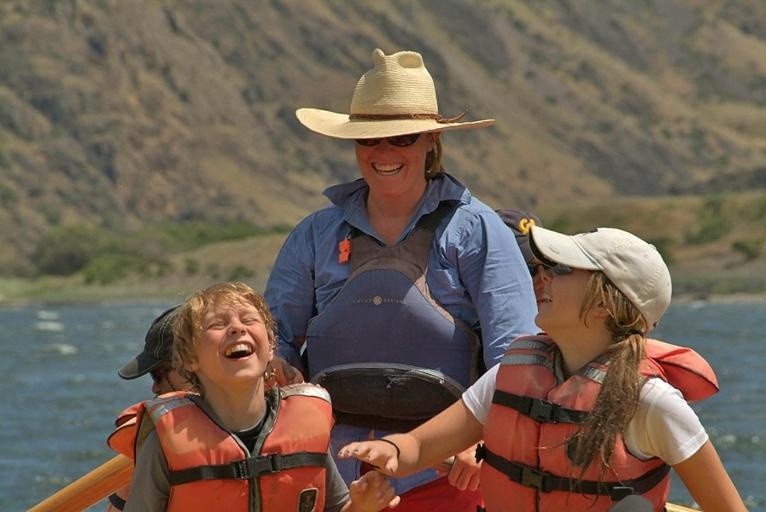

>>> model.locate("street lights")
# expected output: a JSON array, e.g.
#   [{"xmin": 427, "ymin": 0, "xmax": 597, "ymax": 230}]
[{"xmin": 495, "ymin": 209, "xmax": 544, "ymax": 261}]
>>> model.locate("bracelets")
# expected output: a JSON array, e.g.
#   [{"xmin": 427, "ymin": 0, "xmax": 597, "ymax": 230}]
[{"xmin": 375, "ymin": 438, "xmax": 400, "ymax": 458}]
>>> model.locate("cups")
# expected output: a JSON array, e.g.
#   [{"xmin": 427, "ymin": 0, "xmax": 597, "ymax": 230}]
[
  {"xmin": 150, "ymin": 372, "xmax": 163, "ymax": 385},
  {"xmin": 553, "ymin": 265, "xmax": 574, "ymax": 280},
  {"xmin": 356, "ymin": 134, "xmax": 420, "ymax": 146}
]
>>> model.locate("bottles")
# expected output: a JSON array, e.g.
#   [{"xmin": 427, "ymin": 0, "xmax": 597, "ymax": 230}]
[
  {"xmin": 295, "ymin": 48, "xmax": 496, "ymax": 143},
  {"xmin": 117, "ymin": 303, "xmax": 184, "ymax": 379},
  {"xmin": 529, "ymin": 224, "xmax": 672, "ymax": 335}
]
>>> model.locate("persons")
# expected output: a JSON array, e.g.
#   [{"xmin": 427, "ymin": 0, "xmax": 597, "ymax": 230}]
[
  {"xmin": 107, "ymin": 303, "xmax": 202, "ymax": 512},
  {"xmin": 337, "ymin": 224, "xmax": 750, "ymax": 512},
  {"xmin": 123, "ymin": 281, "xmax": 401, "ymax": 511},
  {"xmin": 259, "ymin": 50, "xmax": 544, "ymax": 512}
]
